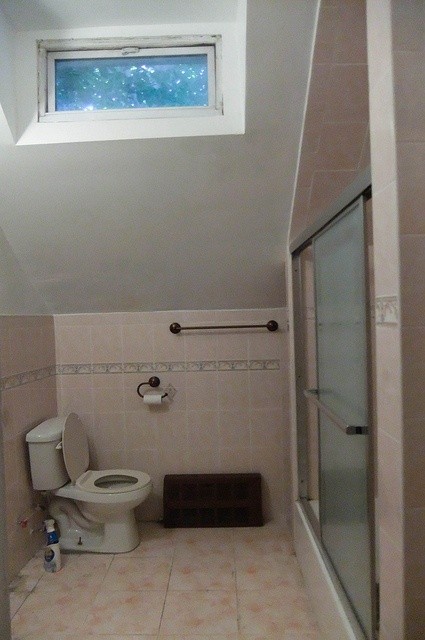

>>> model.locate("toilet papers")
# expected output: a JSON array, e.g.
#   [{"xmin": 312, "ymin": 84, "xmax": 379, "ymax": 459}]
[{"xmin": 143, "ymin": 394, "xmax": 163, "ymax": 405}]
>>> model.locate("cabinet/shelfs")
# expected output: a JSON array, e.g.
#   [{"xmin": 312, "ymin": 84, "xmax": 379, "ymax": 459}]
[{"xmin": 161, "ymin": 472, "xmax": 264, "ymax": 529}]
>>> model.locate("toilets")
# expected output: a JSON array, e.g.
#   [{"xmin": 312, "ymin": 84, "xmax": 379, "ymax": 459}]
[{"xmin": 26, "ymin": 412, "xmax": 152, "ymax": 554}]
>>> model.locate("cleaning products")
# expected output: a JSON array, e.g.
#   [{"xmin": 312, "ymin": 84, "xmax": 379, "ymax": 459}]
[{"xmin": 42, "ymin": 519, "xmax": 61, "ymax": 572}]
